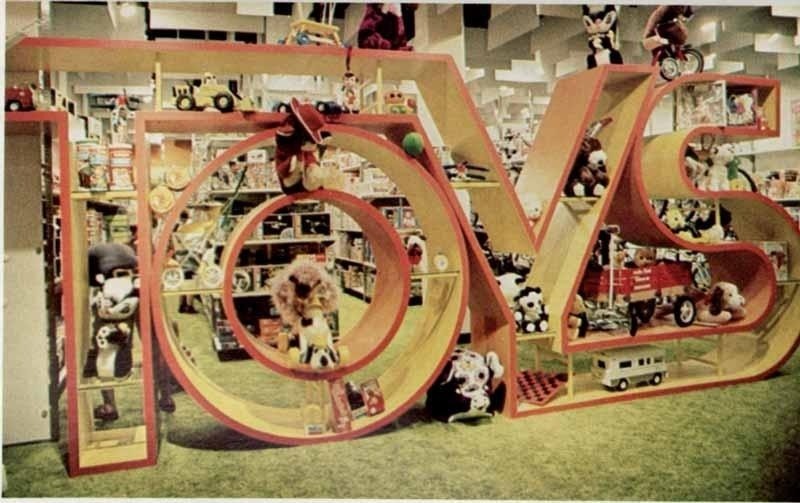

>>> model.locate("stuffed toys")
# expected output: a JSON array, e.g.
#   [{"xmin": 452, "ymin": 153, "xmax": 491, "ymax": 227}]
[
  {"xmin": 271, "ymin": 259, "xmax": 341, "ymax": 371},
  {"xmin": 562, "ymin": 117, "xmax": 613, "ymax": 198},
  {"xmin": 581, "ymin": 2, "xmax": 622, "ymax": 68},
  {"xmin": 683, "ymin": 133, "xmax": 734, "ymax": 191},
  {"xmin": 727, "ymin": 92, "xmax": 754, "ymax": 126},
  {"xmin": 358, "ymin": 4, "xmax": 417, "ymax": 55},
  {"xmin": 420, "ymin": 348, "xmax": 506, "ymax": 423},
  {"xmin": 567, "ymin": 225, "xmax": 748, "ymax": 340},
  {"xmin": 82, "ymin": 242, "xmax": 139, "ymax": 380},
  {"xmin": 276, "ymin": 97, "xmax": 331, "ymax": 193},
  {"xmin": 472, "ymin": 212, "xmax": 550, "ymax": 334},
  {"xmin": 650, "ymin": 196, "xmax": 739, "ymax": 243}
]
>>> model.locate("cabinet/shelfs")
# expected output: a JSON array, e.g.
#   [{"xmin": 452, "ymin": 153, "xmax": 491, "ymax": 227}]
[{"xmin": 5, "ymin": 34, "xmax": 799, "ymax": 472}]
